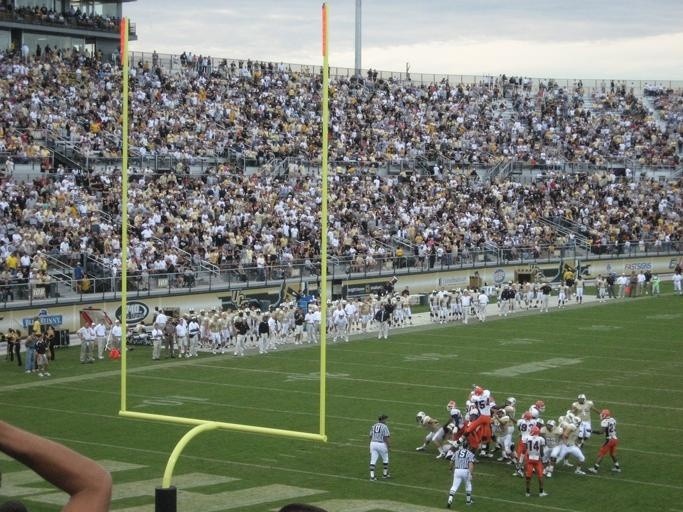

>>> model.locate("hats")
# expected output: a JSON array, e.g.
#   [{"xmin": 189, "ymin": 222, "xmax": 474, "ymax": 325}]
[
  {"xmin": 462, "ymin": 440, "xmax": 470, "ymax": 449},
  {"xmin": 378, "ymin": 415, "xmax": 388, "ymax": 421}
]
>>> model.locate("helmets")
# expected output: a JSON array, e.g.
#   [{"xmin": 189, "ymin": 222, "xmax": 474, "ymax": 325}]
[{"xmin": 416, "ymin": 385, "xmax": 612, "ymax": 435}]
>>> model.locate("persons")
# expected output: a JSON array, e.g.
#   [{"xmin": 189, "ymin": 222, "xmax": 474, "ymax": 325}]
[
  {"xmin": 326, "ymin": 295, "xmax": 414, "ymax": 343},
  {"xmin": 128, "ymin": 297, "xmax": 321, "ymax": 361},
  {"xmin": 427, "ymin": 268, "xmax": 652, "ymax": 325},
  {"xmin": 0, "ymin": 39, "xmax": 683, "ymax": 295},
  {"xmin": 414, "ymin": 385, "xmax": 622, "ymax": 506},
  {"xmin": 366, "ymin": 414, "xmax": 392, "ymax": 483},
  {"xmin": 0, "ymin": 0, "xmax": 120, "ymax": 33},
  {"xmin": 2, "ymin": 316, "xmax": 123, "ymax": 377}
]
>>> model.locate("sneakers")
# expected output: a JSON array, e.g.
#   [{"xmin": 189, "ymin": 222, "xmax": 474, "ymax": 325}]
[
  {"xmin": 445, "ymin": 439, "xmax": 622, "ymax": 478},
  {"xmin": 151, "ymin": 322, "xmax": 414, "ymax": 360},
  {"xmin": 415, "ymin": 446, "xmax": 427, "ymax": 451},
  {"xmin": 539, "ymin": 490, "xmax": 548, "ymax": 497},
  {"xmin": 447, "ymin": 499, "xmax": 452, "ymax": 508},
  {"xmin": 25, "ymin": 367, "xmax": 51, "ymax": 377},
  {"xmin": 382, "ymin": 472, "xmax": 391, "ymax": 478},
  {"xmin": 369, "ymin": 477, "xmax": 377, "ymax": 482},
  {"xmin": 526, "ymin": 492, "xmax": 531, "ymax": 496},
  {"xmin": 436, "ymin": 452, "xmax": 446, "ymax": 459},
  {"xmin": 465, "ymin": 500, "xmax": 475, "ymax": 505}
]
